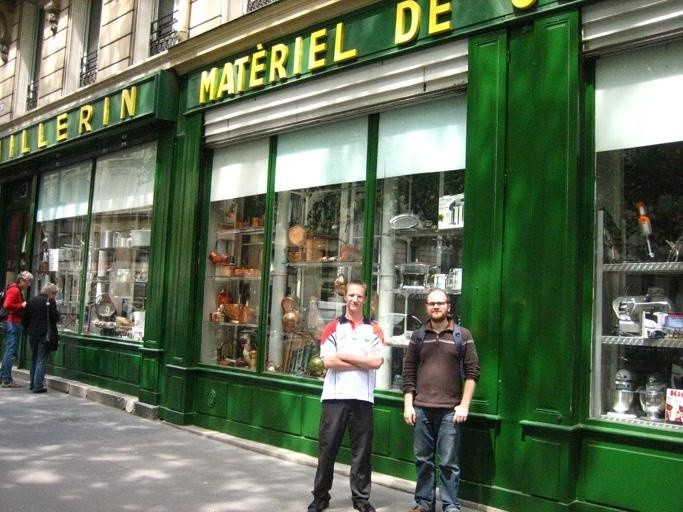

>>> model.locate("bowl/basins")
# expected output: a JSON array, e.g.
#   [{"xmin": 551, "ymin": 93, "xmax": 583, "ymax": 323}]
[{"xmin": 220, "ymin": 302, "xmax": 247, "ymax": 322}]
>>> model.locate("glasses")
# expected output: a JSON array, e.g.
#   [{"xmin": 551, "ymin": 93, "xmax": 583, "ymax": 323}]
[{"xmin": 426, "ymin": 300, "xmax": 448, "ymax": 306}]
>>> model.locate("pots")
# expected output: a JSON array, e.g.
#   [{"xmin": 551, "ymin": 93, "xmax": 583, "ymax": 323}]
[
  {"xmin": 450, "ymin": 198, "xmax": 464, "ymax": 224},
  {"xmin": 395, "ymin": 259, "xmax": 461, "ymax": 292},
  {"xmin": 99, "ymin": 227, "xmax": 150, "ymax": 247}
]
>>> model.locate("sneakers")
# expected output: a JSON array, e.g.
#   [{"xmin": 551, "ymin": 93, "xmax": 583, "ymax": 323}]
[
  {"xmin": 410, "ymin": 504, "xmax": 426, "ymax": 512},
  {"xmin": 29, "ymin": 387, "xmax": 48, "ymax": 392},
  {"xmin": 352, "ymin": 501, "xmax": 375, "ymax": 512},
  {"xmin": 444, "ymin": 506, "xmax": 460, "ymax": 512},
  {"xmin": 0, "ymin": 380, "xmax": 22, "ymax": 387},
  {"xmin": 308, "ymin": 497, "xmax": 329, "ymax": 512}
]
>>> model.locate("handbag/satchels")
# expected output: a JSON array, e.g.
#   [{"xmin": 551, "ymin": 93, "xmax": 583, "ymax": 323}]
[
  {"xmin": 44, "ymin": 329, "xmax": 58, "ymax": 351},
  {"xmin": 0, "ymin": 290, "xmax": 8, "ymax": 319}
]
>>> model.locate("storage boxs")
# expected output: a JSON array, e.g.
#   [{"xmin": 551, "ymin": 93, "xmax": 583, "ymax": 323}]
[
  {"xmin": 130, "ymin": 229, "xmax": 151, "ymax": 245},
  {"xmin": 48, "ymin": 248, "xmax": 72, "ymax": 272}
]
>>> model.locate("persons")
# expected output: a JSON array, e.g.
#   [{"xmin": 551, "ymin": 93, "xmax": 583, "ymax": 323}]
[
  {"xmin": 20, "ymin": 283, "xmax": 60, "ymax": 393},
  {"xmin": 402, "ymin": 288, "xmax": 480, "ymax": 512},
  {"xmin": 307, "ymin": 277, "xmax": 386, "ymax": 511},
  {"xmin": 1, "ymin": 270, "xmax": 35, "ymax": 388}
]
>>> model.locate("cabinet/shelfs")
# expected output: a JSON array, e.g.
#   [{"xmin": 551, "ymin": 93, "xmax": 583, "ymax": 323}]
[
  {"xmin": 208, "ymin": 207, "xmax": 462, "ymax": 394},
  {"xmin": 594, "ymin": 210, "xmax": 683, "ymax": 433},
  {"xmin": 36, "ymin": 245, "xmax": 149, "ymax": 337}
]
